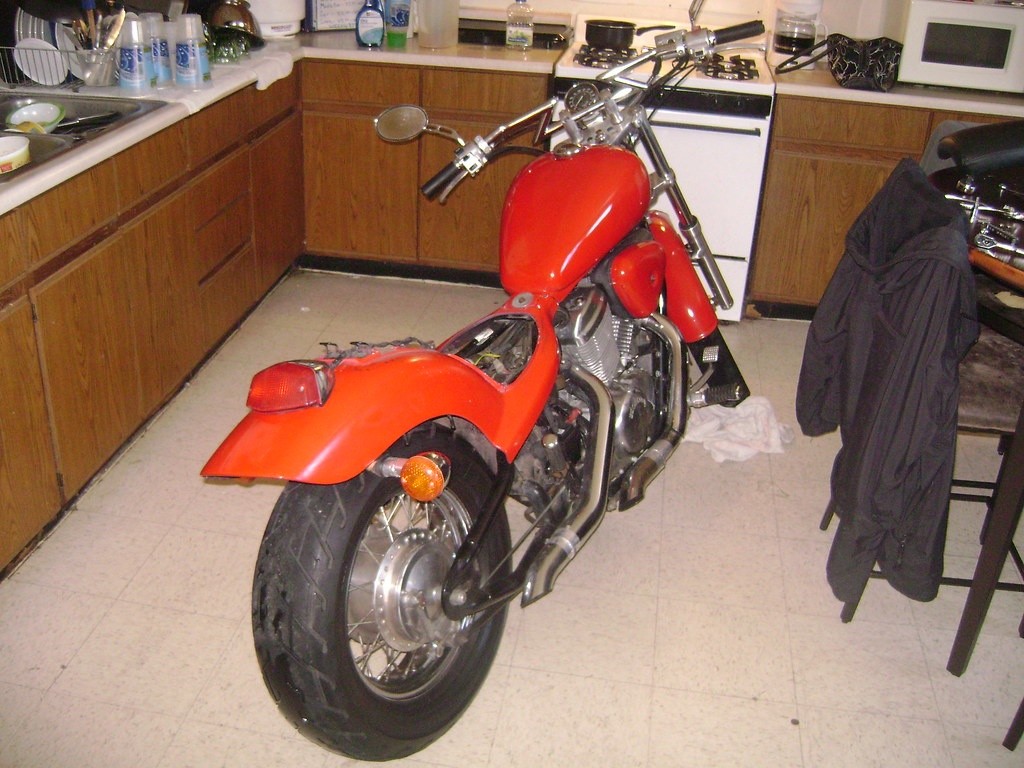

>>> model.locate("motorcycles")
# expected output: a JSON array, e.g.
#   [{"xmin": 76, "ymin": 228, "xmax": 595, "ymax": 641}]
[{"xmin": 198, "ymin": 18, "xmax": 770, "ymax": 765}]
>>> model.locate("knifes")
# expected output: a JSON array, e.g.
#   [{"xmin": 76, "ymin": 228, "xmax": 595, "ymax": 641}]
[{"xmin": 62, "ymin": 8, "xmax": 126, "ymax": 85}]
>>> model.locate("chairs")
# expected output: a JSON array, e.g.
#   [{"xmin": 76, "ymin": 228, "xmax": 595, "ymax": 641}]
[{"xmin": 820, "ymin": 154, "xmax": 1024, "ymax": 642}]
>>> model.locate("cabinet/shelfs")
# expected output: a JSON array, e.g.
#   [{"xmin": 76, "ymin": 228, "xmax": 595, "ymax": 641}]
[
  {"xmin": 745, "ymin": 95, "xmax": 1024, "ymax": 320},
  {"xmin": 306, "ymin": 58, "xmax": 551, "ymax": 288},
  {"xmin": 0, "ymin": 62, "xmax": 306, "ymax": 578}
]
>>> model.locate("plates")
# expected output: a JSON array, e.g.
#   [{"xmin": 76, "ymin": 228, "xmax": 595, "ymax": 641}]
[{"xmin": 15, "ymin": 7, "xmax": 83, "ymax": 86}]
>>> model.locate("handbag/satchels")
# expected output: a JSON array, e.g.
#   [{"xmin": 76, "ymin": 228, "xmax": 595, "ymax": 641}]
[{"xmin": 775, "ymin": 34, "xmax": 904, "ymax": 92}]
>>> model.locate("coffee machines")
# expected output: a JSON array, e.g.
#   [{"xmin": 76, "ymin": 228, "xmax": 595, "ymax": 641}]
[{"xmin": 766, "ymin": 0, "xmax": 828, "ymax": 69}]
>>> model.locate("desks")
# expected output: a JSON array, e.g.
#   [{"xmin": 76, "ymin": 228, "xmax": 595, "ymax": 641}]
[{"xmin": 928, "ymin": 166, "xmax": 1024, "ymax": 676}]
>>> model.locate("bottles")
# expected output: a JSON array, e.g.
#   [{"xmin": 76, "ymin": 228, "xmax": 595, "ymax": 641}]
[{"xmin": 506, "ymin": 0, "xmax": 534, "ymax": 52}]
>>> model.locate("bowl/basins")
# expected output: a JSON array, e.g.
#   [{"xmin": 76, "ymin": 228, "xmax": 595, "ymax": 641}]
[
  {"xmin": 5, "ymin": 102, "xmax": 66, "ymax": 133},
  {"xmin": 0, "ymin": 136, "xmax": 31, "ymax": 174},
  {"xmin": 205, "ymin": 0, "xmax": 265, "ymax": 48}
]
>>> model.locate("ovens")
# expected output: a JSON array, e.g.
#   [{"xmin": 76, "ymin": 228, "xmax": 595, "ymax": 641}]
[{"xmin": 550, "ymin": 102, "xmax": 771, "ymax": 322}]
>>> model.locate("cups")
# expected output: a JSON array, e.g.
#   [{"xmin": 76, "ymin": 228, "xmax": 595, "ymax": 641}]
[
  {"xmin": 418, "ymin": 0, "xmax": 459, "ymax": 49},
  {"xmin": 119, "ymin": 12, "xmax": 212, "ymax": 95},
  {"xmin": 76, "ymin": 50, "xmax": 116, "ymax": 86},
  {"xmin": 385, "ymin": 0, "xmax": 411, "ymax": 47}
]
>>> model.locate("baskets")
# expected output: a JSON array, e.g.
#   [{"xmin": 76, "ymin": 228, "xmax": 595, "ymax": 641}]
[{"xmin": 0, "ymin": 47, "xmax": 116, "ymax": 93}]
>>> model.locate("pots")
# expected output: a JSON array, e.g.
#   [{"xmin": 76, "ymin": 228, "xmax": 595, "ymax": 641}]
[{"xmin": 585, "ymin": 18, "xmax": 675, "ymax": 50}]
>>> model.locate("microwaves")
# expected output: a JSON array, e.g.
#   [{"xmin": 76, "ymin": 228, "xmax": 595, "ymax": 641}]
[{"xmin": 882, "ymin": 0, "xmax": 1024, "ymax": 93}]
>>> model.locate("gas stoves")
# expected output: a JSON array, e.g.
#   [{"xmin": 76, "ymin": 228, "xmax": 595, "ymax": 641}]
[{"xmin": 555, "ymin": 11, "xmax": 776, "ymax": 118}]
